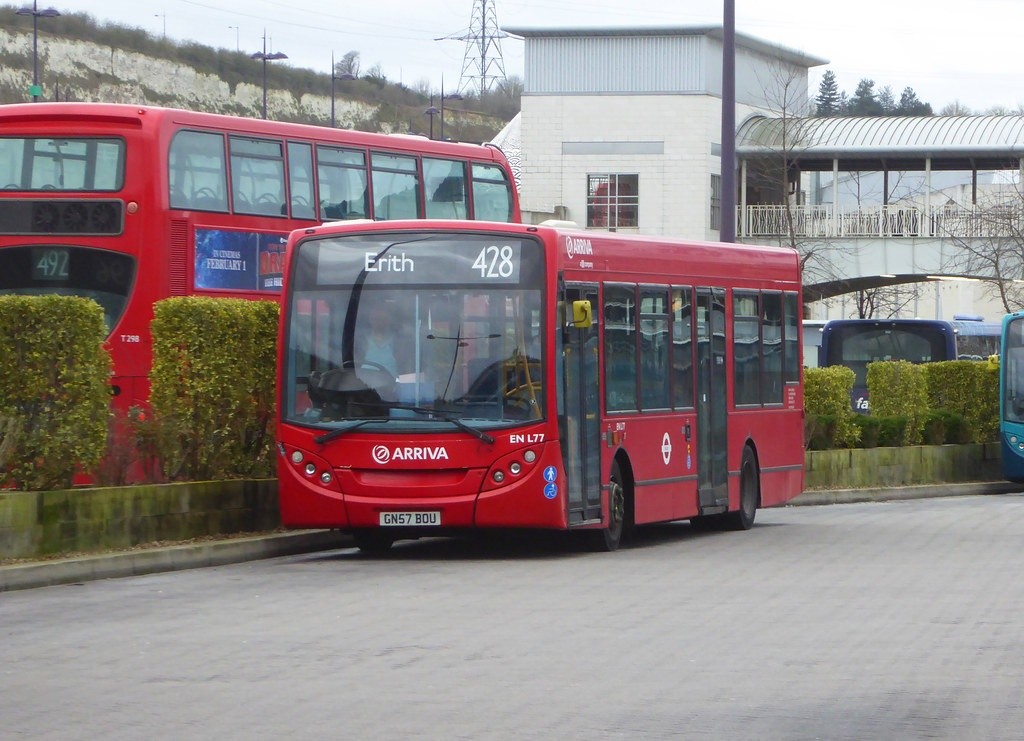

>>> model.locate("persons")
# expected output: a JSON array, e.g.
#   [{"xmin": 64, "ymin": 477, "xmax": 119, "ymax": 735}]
[{"xmin": 354, "ymin": 305, "xmax": 418, "ymax": 373}]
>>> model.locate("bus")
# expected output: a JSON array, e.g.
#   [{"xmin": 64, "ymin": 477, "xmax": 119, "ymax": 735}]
[
  {"xmin": 0, "ymin": 102, "xmax": 522, "ymax": 491},
  {"xmin": 819, "ymin": 315, "xmax": 1020, "ymax": 414},
  {"xmin": 276, "ymin": 220, "xmax": 806, "ymax": 552},
  {"xmin": 1000, "ymin": 311, "xmax": 1024, "ymax": 483}
]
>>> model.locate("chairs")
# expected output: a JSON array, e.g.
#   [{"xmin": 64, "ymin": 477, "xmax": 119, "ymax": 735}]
[{"xmin": 170, "ymin": 184, "xmax": 327, "ymax": 218}]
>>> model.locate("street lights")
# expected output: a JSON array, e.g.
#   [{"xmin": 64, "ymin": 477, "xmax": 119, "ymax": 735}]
[
  {"xmin": 423, "ymin": 88, "xmax": 440, "ymax": 140},
  {"xmin": 15, "ymin": 0, "xmax": 61, "ymax": 103},
  {"xmin": 261, "ymin": 37, "xmax": 272, "ymax": 65},
  {"xmin": 155, "ymin": 14, "xmax": 165, "ymax": 38},
  {"xmin": 250, "ymin": 27, "xmax": 288, "ymax": 120},
  {"xmin": 229, "ymin": 26, "xmax": 239, "ymax": 53}
]
